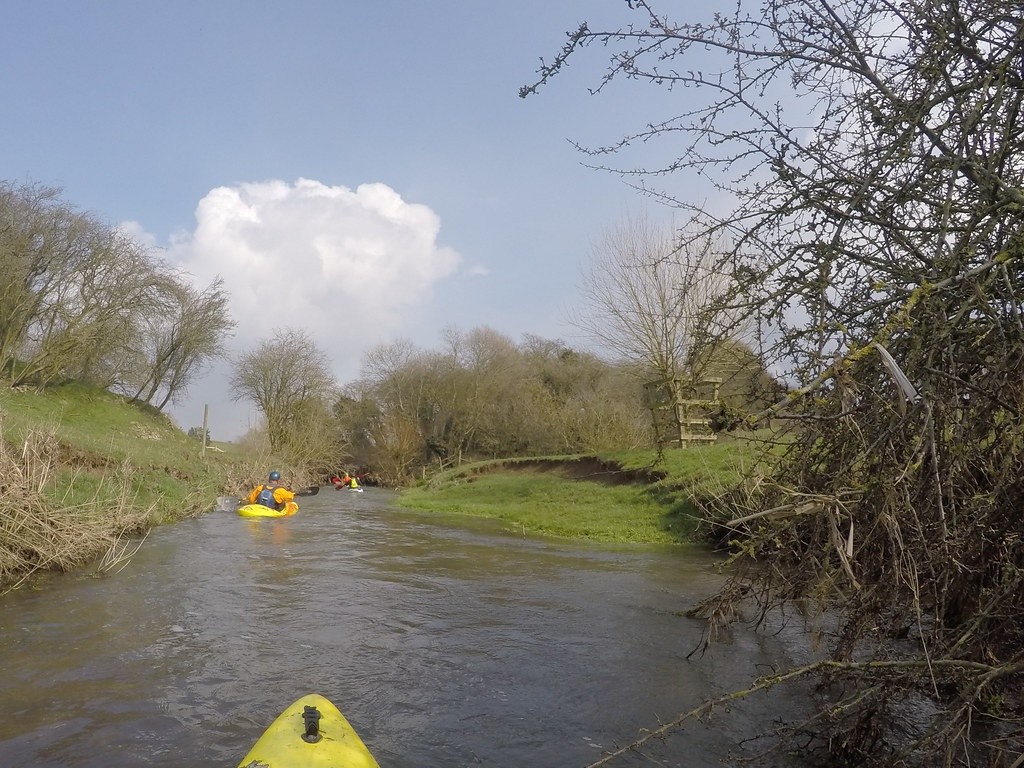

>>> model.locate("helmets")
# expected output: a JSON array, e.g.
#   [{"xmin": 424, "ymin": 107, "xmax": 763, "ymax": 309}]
[{"xmin": 270, "ymin": 472, "xmax": 280, "ymax": 480}]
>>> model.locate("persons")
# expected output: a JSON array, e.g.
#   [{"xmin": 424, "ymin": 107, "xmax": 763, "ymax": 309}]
[
  {"xmin": 249, "ymin": 471, "xmax": 295, "ymax": 512},
  {"xmin": 349, "ymin": 475, "xmax": 360, "ymax": 488}
]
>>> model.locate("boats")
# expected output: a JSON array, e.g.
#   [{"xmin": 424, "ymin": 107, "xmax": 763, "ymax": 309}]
[
  {"xmin": 237, "ymin": 501, "xmax": 300, "ymax": 519},
  {"xmin": 348, "ymin": 485, "xmax": 363, "ymax": 493}
]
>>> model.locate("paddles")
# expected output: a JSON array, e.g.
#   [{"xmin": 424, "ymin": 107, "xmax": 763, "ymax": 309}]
[
  {"xmin": 237, "ymin": 486, "xmax": 320, "ymax": 507},
  {"xmin": 334, "ymin": 472, "xmax": 369, "ymax": 491}
]
